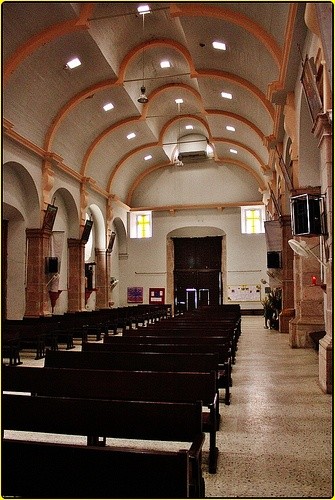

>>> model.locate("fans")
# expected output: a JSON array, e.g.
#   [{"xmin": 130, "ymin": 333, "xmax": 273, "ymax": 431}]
[{"xmin": 288, "ymin": 239, "xmax": 330, "ymax": 274}]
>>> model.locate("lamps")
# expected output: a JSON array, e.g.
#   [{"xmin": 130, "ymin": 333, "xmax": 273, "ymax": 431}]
[
  {"xmin": 138, "ymin": 14, "xmax": 148, "ymax": 103},
  {"xmin": 175, "ymin": 122, "xmax": 185, "ymax": 167}
]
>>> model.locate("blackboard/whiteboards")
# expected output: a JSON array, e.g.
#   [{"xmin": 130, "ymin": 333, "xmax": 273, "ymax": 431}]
[{"xmin": 226, "ymin": 284, "xmax": 261, "ymax": 302}]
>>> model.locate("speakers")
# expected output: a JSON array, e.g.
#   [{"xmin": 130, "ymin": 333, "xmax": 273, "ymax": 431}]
[
  {"xmin": 46, "ymin": 259, "xmax": 58, "ymax": 273},
  {"xmin": 267, "ymin": 251, "xmax": 280, "ymax": 268},
  {"xmin": 294, "ymin": 200, "xmax": 321, "ymax": 237}
]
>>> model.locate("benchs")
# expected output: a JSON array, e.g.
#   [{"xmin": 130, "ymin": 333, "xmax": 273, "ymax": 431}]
[{"xmin": 1, "ymin": 305, "xmax": 241, "ymax": 499}]
